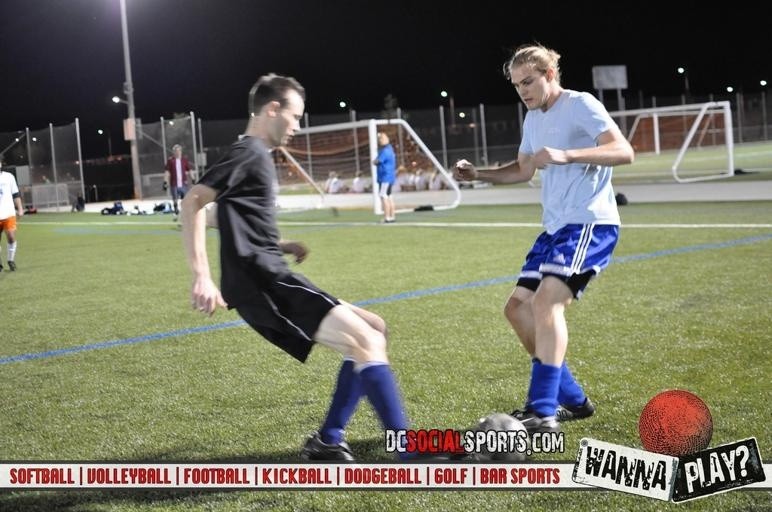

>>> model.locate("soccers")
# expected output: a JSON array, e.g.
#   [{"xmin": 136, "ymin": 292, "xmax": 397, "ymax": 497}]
[{"xmin": 466, "ymin": 412, "xmax": 531, "ymax": 464}]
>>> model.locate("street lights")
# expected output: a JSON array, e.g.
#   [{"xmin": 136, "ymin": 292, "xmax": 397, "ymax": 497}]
[{"xmin": 112, "ymin": 96, "xmax": 143, "ymax": 200}]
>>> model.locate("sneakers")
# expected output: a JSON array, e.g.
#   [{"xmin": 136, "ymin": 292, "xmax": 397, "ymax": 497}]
[
  {"xmin": 509, "ymin": 409, "xmax": 560, "ymax": 437},
  {"xmin": 407, "ymin": 447, "xmax": 469, "ymax": 462},
  {"xmin": 0, "ymin": 261, "xmax": 18, "ymax": 273},
  {"xmin": 299, "ymin": 429, "xmax": 357, "ymax": 464},
  {"xmin": 553, "ymin": 397, "xmax": 595, "ymax": 422}
]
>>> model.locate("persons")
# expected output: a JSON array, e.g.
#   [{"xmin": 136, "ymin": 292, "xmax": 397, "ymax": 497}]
[
  {"xmin": 450, "ymin": 43, "xmax": 635, "ymax": 439},
  {"xmin": 178, "ymin": 72, "xmax": 470, "ymax": 463},
  {"xmin": 0, "ymin": 152, "xmax": 25, "ymax": 273},
  {"xmin": 323, "ymin": 165, "xmax": 450, "ymax": 194},
  {"xmin": 26, "ymin": 204, "xmax": 38, "ymax": 215},
  {"xmin": 70, "ymin": 192, "xmax": 85, "ymax": 212},
  {"xmin": 372, "ymin": 130, "xmax": 398, "ymax": 225},
  {"xmin": 162, "ymin": 144, "xmax": 196, "ymax": 222}
]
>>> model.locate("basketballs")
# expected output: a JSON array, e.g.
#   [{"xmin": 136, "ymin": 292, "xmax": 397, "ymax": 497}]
[{"xmin": 639, "ymin": 390, "xmax": 713, "ymax": 457}]
